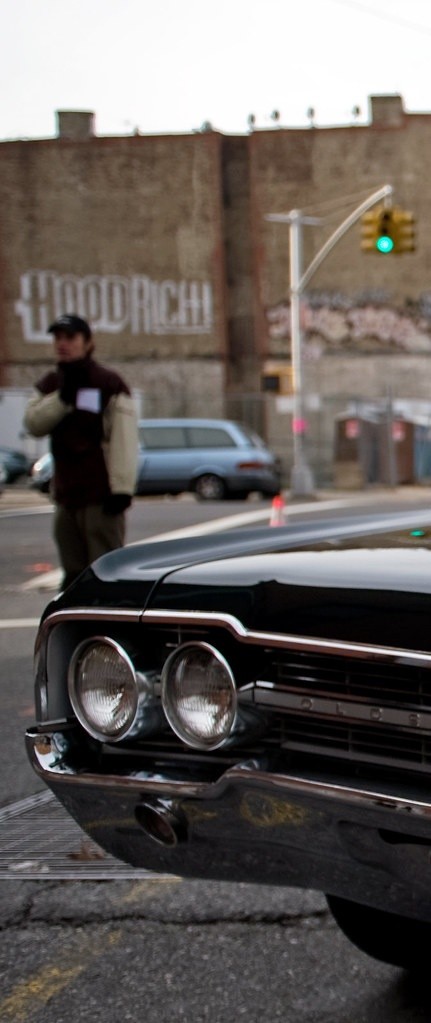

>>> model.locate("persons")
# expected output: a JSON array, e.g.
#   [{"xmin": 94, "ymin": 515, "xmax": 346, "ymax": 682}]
[{"xmin": 21, "ymin": 315, "xmax": 140, "ymax": 595}]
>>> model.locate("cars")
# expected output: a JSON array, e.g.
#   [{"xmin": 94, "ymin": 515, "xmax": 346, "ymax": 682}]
[
  {"xmin": 24, "ymin": 507, "xmax": 430, "ymax": 976},
  {"xmin": 0, "ymin": 445, "xmax": 32, "ymax": 487},
  {"xmin": 29, "ymin": 415, "xmax": 285, "ymax": 503}
]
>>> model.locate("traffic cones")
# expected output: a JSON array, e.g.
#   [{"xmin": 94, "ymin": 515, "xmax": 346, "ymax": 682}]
[{"xmin": 266, "ymin": 495, "xmax": 287, "ymax": 526}]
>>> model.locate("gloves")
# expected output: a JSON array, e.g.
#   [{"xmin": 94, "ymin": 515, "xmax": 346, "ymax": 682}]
[
  {"xmin": 58, "ymin": 366, "xmax": 86, "ymax": 405},
  {"xmin": 102, "ymin": 493, "xmax": 134, "ymax": 517}
]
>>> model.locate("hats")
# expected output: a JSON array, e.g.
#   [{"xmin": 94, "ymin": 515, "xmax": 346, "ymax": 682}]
[{"xmin": 47, "ymin": 315, "xmax": 91, "ymax": 339}]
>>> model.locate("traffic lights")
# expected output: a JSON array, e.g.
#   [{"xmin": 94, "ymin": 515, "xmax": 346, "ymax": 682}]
[
  {"xmin": 358, "ymin": 205, "xmax": 371, "ymax": 256},
  {"xmin": 375, "ymin": 207, "xmax": 396, "ymax": 254},
  {"xmin": 395, "ymin": 209, "xmax": 417, "ymax": 254}
]
